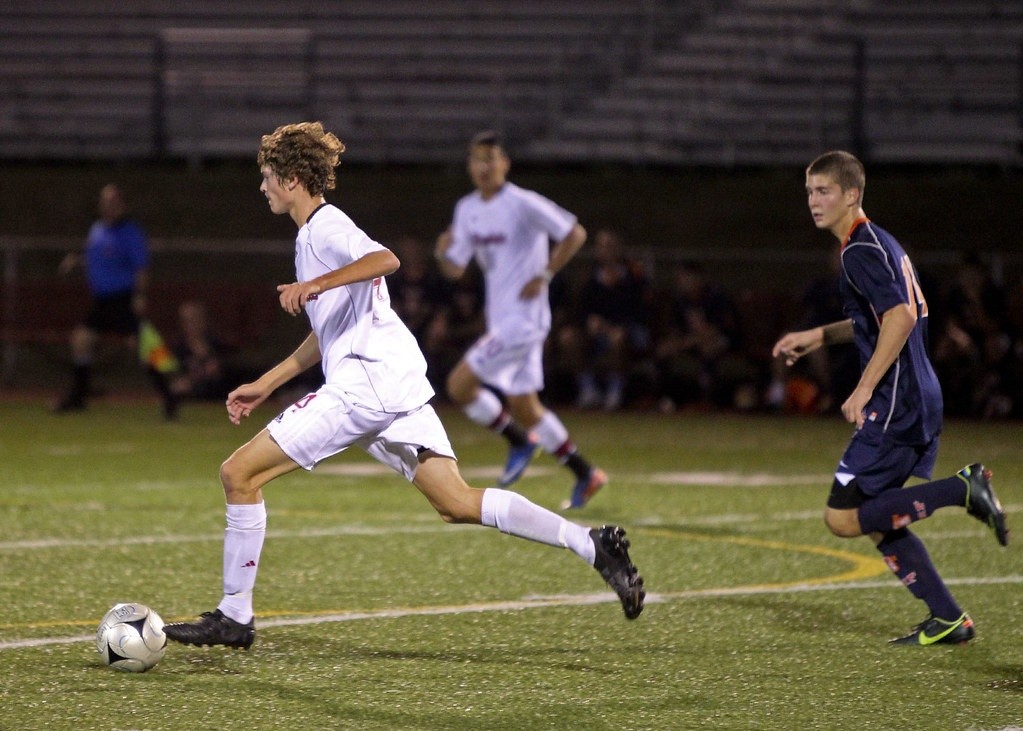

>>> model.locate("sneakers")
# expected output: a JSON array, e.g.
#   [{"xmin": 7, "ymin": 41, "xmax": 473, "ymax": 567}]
[
  {"xmin": 563, "ymin": 467, "xmax": 607, "ymax": 509},
  {"xmin": 589, "ymin": 526, "xmax": 645, "ymax": 619},
  {"xmin": 888, "ymin": 612, "xmax": 977, "ymax": 646},
  {"xmin": 161, "ymin": 609, "xmax": 256, "ymax": 651},
  {"xmin": 955, "ymin": 463, "xmax": 1010, "ymax": 547},
  {"xmin": 497, "ymin": 433, "xmax": 543, "ymax": 488}
]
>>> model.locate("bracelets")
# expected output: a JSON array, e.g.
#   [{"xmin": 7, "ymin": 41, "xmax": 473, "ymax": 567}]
[{"xmin": 537, "ymin": 268, "xmax": 553, "ymax": 284}]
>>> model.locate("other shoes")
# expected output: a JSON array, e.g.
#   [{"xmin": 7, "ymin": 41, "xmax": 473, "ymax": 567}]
[
  {"xmin": 163, "ymin": 397, "xmax": 179, "ymax": 420},
  {"xmin": 52, "ymin": 398, "xmax": 87, "ymax": 415}
]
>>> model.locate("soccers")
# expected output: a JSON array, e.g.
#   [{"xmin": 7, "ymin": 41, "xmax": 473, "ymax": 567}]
[{"xmin": 96, "ymin": 602, "xmax": 168, "ymax": 672}]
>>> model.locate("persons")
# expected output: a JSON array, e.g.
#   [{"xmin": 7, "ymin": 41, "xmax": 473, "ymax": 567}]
[
  {"xmin": 773, "ymin": 153, "xmax": 1007, "ymax": 647},
  {"xmin": 164, "ymin": 123, "xmax": 646, "ymax": 649},
  {"xmin": 49, "ymin": 186, "xmax": 1023, "ymax": 420},
  {"xmin": 437, "ymin": 132, "xmax": 608, "ymax": 511}
]
